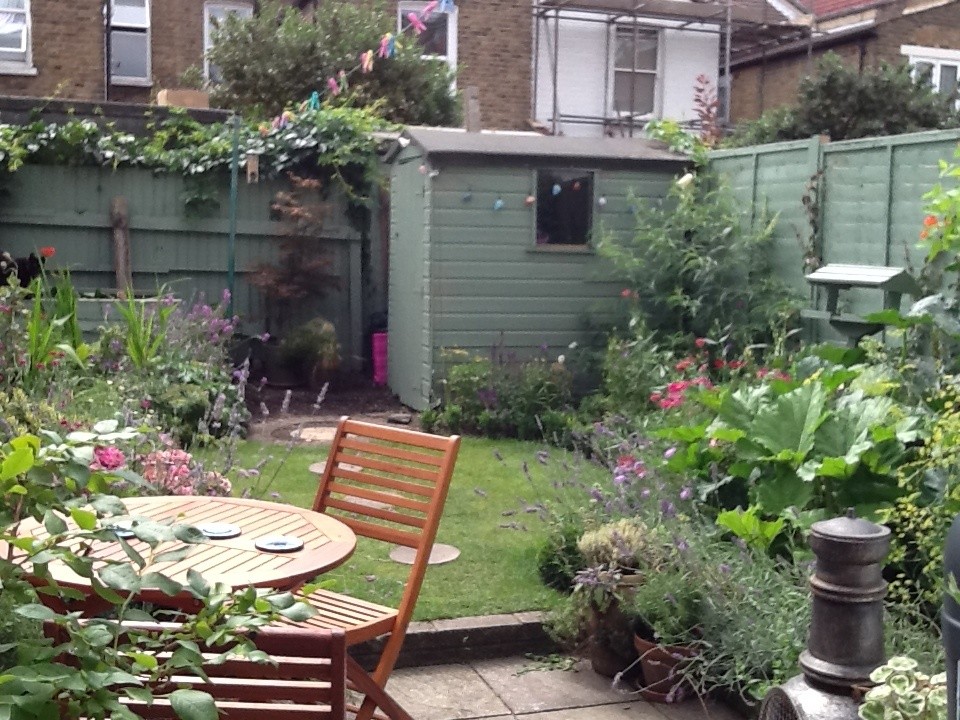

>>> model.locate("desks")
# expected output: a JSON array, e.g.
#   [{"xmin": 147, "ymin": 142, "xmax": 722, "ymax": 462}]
[{"xmin": 0, "ymin": 495, "xmax": 357, "ymax": 619}]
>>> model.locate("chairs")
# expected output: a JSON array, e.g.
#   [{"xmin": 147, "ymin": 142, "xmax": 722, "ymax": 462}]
[{"xmin": 41, "ymin": 415, "xmax": 462, "ymax": 720}]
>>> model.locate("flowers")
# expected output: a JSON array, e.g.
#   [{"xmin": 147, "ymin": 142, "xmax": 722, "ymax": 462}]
[{"xmin": 473, "ymin": 415, "xmax": 817, "ymax": 720}]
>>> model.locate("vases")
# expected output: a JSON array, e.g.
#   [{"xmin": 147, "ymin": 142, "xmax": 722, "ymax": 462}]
[
  {"xmin": 589, "ymin": 561, "xmax": 648, "ymax": 678},
  {"xmin": 633, "ymin": 627, "xmax": 704, "ymax": 694}
]
